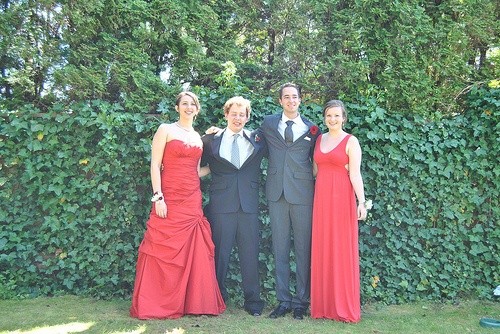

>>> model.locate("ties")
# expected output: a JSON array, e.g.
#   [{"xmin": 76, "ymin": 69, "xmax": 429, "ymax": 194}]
[
  {"xmin": 285, "ymin": 120, "xmax": 294, "ymax": 146},
  {"xmin": 230, "ymin": 134, "xmax": 240, "ymax": 169}
]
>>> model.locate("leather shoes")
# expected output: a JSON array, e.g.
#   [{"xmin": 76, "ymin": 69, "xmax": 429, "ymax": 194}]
[
  {"xmin": 294, "ymin": 307, "xmax": 306, "ymax": 320},
  {"xmin": 269, "ymin": 305, "xmax": 291, "ymax": 319},
  {"xmin": 251, "ymin": 312, "xmax": 260, "ymax": 316}
]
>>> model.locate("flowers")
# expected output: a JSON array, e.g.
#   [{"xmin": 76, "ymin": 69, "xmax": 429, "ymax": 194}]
[
  {"xmin": 255, "ymin": 135, "xmax": 261, "ymax": 142},
  {"xmin": 310, "ymin": 126, "xmax": 319, "ymax": 135}
]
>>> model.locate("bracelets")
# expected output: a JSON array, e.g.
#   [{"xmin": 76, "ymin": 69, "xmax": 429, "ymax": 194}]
[
  {"xmin": 151, "ymin": 193, "xmax": 163, "ymax": 202},
  {"xmin": 359, "ymin": 200, "xmax": 372, "ymax": 210}
]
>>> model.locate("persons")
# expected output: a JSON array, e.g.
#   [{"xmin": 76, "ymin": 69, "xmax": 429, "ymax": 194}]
[
  {"xmin": 160, "ymin": 96, "xmax": 269, "ymax": 315},
  {"xmin": 130, "ymin": 92, "xmax": 226, "ymax": 319},
  {"xmin": 310, "ymin": 99, "xmax": 367, "ymax": 322},
  {"xmin": 206, "ymin": 82, "xmax": 322, "ymax": 320}
]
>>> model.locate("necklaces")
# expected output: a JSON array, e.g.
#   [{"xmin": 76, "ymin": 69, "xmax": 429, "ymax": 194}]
[{"xmin": 176, "ymin": 122, "xmax": 194, "ymax": 133}]
